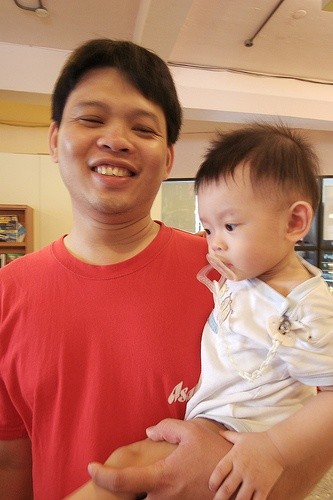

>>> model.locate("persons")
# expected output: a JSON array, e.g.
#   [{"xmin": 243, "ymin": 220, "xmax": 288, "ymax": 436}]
[
  {"xmin": 1, "ymin": 37, "xmax": 225, "ymax": 500},
  {"xmin": 67, "ymin": 114, "xmax": 333, "ymax": 500}
]
